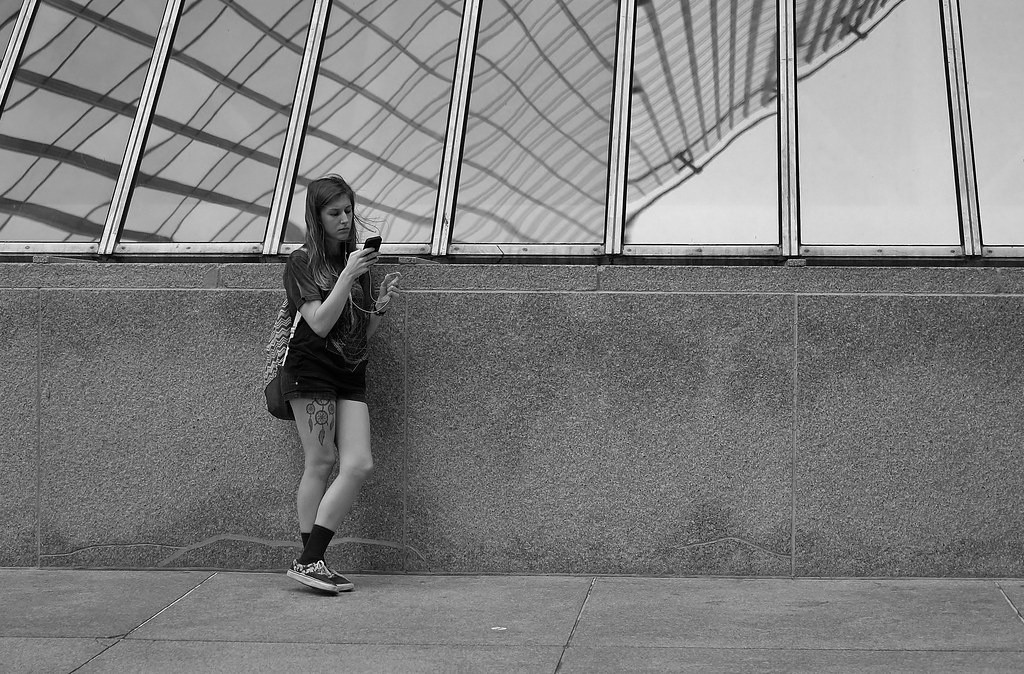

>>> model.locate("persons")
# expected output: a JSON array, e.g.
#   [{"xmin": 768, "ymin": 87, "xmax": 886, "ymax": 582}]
[{"xmin": 280, "ymin": 173, "xmax": 402, "ymax": 598}]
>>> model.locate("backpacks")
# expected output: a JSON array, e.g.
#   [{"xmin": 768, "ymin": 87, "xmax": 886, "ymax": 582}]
[{"xmin": 263, "ymin": 244, "xmax": 311, "ymax": 420}]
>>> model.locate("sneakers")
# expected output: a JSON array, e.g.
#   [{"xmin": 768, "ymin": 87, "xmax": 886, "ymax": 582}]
[
  {"xmin": 328, "ymin": 568, "xmax": 354, "ymax": 591},
  {"xmin": 287, "ymin": 558, "xmax": 339, "ymax": 592}
]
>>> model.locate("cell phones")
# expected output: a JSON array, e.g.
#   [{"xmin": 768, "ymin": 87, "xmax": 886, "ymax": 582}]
[{"xmin": 363, "ymin": 236, "xmax": 382, "ymax": 254}]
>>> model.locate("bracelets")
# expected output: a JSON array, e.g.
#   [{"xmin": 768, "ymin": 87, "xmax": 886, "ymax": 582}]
[{"xmin": 373, "ymin": 302, "xmax": 386, "ymax": 316}]
